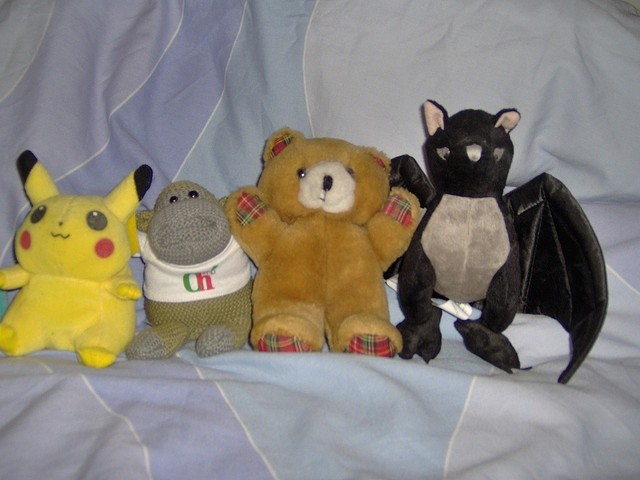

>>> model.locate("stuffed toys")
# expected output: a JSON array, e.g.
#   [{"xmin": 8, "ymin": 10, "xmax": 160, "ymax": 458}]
[
  {"xmin": 383, "ymin": 98, "xmax": 608, "ymax": 386},
  {"xmin": 125, "ymin": 179, "xmax": 252, "ymax": 361},
  {"xmin": 0, "ymin": 149, "xmax": 153, "ymax": 369},
  {"xmin": 222, "ymin": 126, "xmax": 422, "ymax": 358}
]
